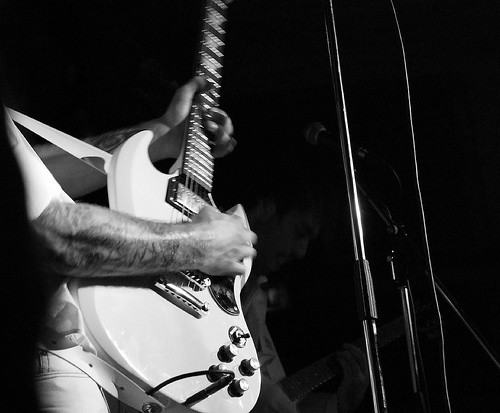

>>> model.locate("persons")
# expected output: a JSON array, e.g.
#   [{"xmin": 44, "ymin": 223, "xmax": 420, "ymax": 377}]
[
  {"xmin": 1, "ymin": 71, "xmax": 261, "ymax": 413},
  {"xmin": 205, "ymin": 169, "xmax": 371, "ymax": 413}
]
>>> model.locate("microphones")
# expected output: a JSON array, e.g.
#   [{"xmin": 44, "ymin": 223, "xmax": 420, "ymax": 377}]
[{"xmin": 303, "ymin": 122, "xmax": 369, "ymax": 158}]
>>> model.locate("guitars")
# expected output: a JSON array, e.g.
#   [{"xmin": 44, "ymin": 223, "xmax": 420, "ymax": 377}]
[{"xmin": 77, "ymin": 1, "xmax": 260, "ymax": 412}]
